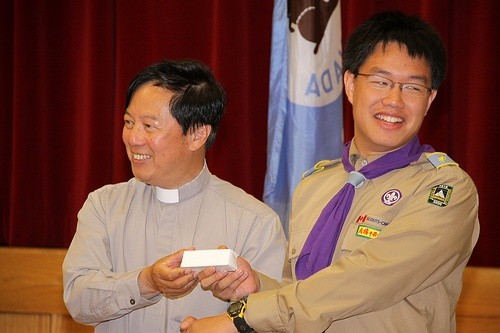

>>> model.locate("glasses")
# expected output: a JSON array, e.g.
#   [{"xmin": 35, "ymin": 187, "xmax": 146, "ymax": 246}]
[{"xmin": 354, "ymin": 73, "xmax": 432, "ymax": 96}]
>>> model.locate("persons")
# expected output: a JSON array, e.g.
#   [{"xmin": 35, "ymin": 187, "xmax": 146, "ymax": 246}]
[
  {"xmin": 61, "ymin": 59, "xmax": 285, "ymax": 333},
  {"xmin": 180, "ymin": 10, "xmax": 481, "ymax": 333}
]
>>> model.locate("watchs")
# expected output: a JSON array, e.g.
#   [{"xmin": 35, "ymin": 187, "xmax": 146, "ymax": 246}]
[{"xmin": 226, "ymin": 296, "xmax": 255, "ymax": 333}]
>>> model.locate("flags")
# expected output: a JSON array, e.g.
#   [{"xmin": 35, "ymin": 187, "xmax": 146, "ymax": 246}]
[{"xmin": 261, "ymin": 1, "xmax": 344, "ymax": 239}]
